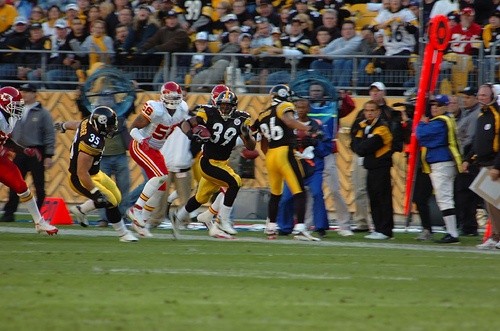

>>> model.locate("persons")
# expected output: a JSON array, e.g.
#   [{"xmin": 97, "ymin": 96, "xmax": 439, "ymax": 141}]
[
  {"xmin": 463, "ymin": 84, "xmax": 500, "ymax": 249},
  {"xmin": 129, "ymin": 81, "xmax": 210, "ymax": 237},
  {"xmin": 350, "ymin": 102, "xmax": 394, "ymax": 240},
  {"xmin": 0, "ymin": 86, "xmax": 58, "ymax": 235},
  {"xmin": 175, "ymin": 92, "xmax": 255, "ymax": 235},
  {"xmin": 0, "ymin": 0, "xmax": 500, "ymax": 250},
  {"xmin": 54, "ymin": 106, "xmax": 139, "ymax": 243},
  {"xmin": 403, "ymin": 95, "xmax": 461, "ymax": 243},
  {"xmin": 250, "ymin": 85, "xmax": 321, "ymax": 242}
]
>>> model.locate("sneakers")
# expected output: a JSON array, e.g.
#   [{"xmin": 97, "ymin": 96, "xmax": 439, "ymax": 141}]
[
  {"xmin": 364, "ymin": 230, "xmax": 392, "ymax": 240},
  {"xmin": 476, "ymin": 239, "xmax": 500, "ymax": 250},
  {"xmin": 73, "ymin": 202, "xmax": 239, "ymax": 242},
  {"xmin": 266, "ymin": 227, "xmax": 277, "ymax": 240},
  {"xmin": 36, "ymin": 217, "xmax": 59, "ymax": 236},
  {"xmin": 435, "ymin": 235, "xmax": 460, "ymax": 245},
  {"xmin": 293, "ymin": 228, "xmax": 321, "ymax": 241}
]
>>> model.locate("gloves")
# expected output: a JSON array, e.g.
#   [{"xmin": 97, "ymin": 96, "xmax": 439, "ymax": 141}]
[
  {"xmin": 192, "ymin": 130, "xmax": 209, "ymax": 144},
  {"xmin": 54, "ymin": 122, "xmax": 66, "ymax": 133},
  {"xmin": 240, "ymin": 124, "xmax": 250, "ymax": 137},
  {"xmin": 24, "ymin": 147, "xmax": 43, "ymax": 161},
  {"xmin": 142, "ymin": 137, "xmax": 153, "ymax": 151}
]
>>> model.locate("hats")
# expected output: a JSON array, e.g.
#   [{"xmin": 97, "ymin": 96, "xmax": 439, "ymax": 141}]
[
  {"xmin": 19, "ymin": 83, "xmax": 37, "ymax": 92},
  {"xmin": 14, "ymin": 3, "xmax": 473, "ymax": 42},
  {"xmin": 429, "ymin": 94, "xmax": 448, "ymax": 104},
  {"xmin": 369, "ymin": 81, "xmax": 385, "ymax": 91},
  {"xmin": 458, "ymin": 87, "xmax": 476, "ymax": 96}
]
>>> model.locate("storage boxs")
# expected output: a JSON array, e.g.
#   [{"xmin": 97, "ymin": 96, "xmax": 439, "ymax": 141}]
[{"xmin": 232, "ymin": 189, "xmax": 269, "ymax": 219}]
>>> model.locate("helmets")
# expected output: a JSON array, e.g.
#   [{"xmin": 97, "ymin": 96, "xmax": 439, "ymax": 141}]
[
  {"xmin": 211, "ymin": 85, "xmax": 229, "ymax": 104},
  {"xmin": 270, "ymin": 85, "xmax": 292, "ymax": 102},
  {"xmin": 0, "ymin": 86, "xmax": 23, "ymax": 120},
  {"xmin": 216, "ymin": 91, "xmax": 238, "ymax": 118},
  {"xmin": 161, "ymin": 82, "xmax": 183, "ymax": 109},
  {"xmin": 90, "ymin": 105, "xmax": 119, "ymax": 139}
]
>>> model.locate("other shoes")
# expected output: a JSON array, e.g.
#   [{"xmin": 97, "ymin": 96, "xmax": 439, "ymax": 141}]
[
  {"xmin": 0, "ymin": 212, "xmax": 14, "ymax": 222},
  {"xmin": 351, "ymin": 224, "xmax": 369, "ymax": 231},
  {"xmin": 339, "ymin": 228, "xmax": 354, "ymax": 236},
  {"xmin": 415, "ymin": 230, "xmax": 431, "ymax": 240}
]
